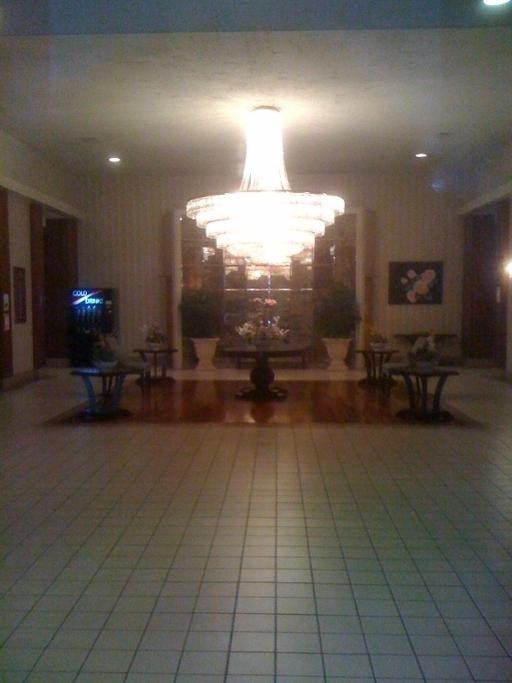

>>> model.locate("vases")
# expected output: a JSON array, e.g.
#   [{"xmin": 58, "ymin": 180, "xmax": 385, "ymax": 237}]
[
  {"xmin": 149, "ymin": 343, "xmax": 159, "ymax": 351},
  {"xmin": 93, "ymin": 360, "xmax": 118, "ymax": 369},
  {"xmin": 416, "ymin": 361, "xmax": 432, "ymax": 369},
  {"xmin": 254, "ymin": 336, "xmax": 271, "ymax": 346}
]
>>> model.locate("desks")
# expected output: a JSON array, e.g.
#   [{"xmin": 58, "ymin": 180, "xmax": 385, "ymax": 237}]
[
  {"xmin": 218, "ymin": 344, "xmax": 306, "ymax": 402},
  {"xmin": 388, "ymin": 365, "xmax": 460, "ymax": 422},
  {"xmin": 74, "ymin": 367, "xmax": 138, "ymax": 422},
  {"xmin": 355, "ymin": 349, "xmax": 399, "ymax": 387},
  {"xmin": 134, "ymin": 349, "xmax": 178, "ymax": 384},
  {"xmin": 398, "ymin": 333, "xmax": 457, "ymax": 364}
]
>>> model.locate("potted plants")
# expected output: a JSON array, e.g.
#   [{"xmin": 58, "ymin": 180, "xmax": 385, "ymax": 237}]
[
  {"xmin": 368, "ymin": 330, "xmax": 386, "ymax": 348},
  {"xmin": 313, "ymin": 281, "xmax": 361, "ymax": 373},
  {"xmin": 179, "ymin": 289, "xmax": 223, "ymax": 373}
]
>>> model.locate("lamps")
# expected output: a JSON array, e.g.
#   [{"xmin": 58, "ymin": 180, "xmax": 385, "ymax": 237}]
[{"xmin": 186, "ymin": 107, "xmax": 347, "ymax": 269}]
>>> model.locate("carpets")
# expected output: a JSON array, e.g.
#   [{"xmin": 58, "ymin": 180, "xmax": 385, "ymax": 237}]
[{"xmin": 49, "ymin": 378, "xmax": 484, "ymax": 429}]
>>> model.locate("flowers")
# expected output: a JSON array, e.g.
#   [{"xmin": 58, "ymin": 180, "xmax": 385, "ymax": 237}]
[
  {"xmin": 409, "ymin": 336, "xmax": 438, "ymax": 362},
  {"xmin": 93, "ymin": 328, "xmax": 120, "ymax": 360},
  {"xmin": 145, "ymin": 319, "xmax": 161, "ymax": 342},
  {"xmin": 234, "ymin": 295, "xmax": 290, "ymax": 341}
]
